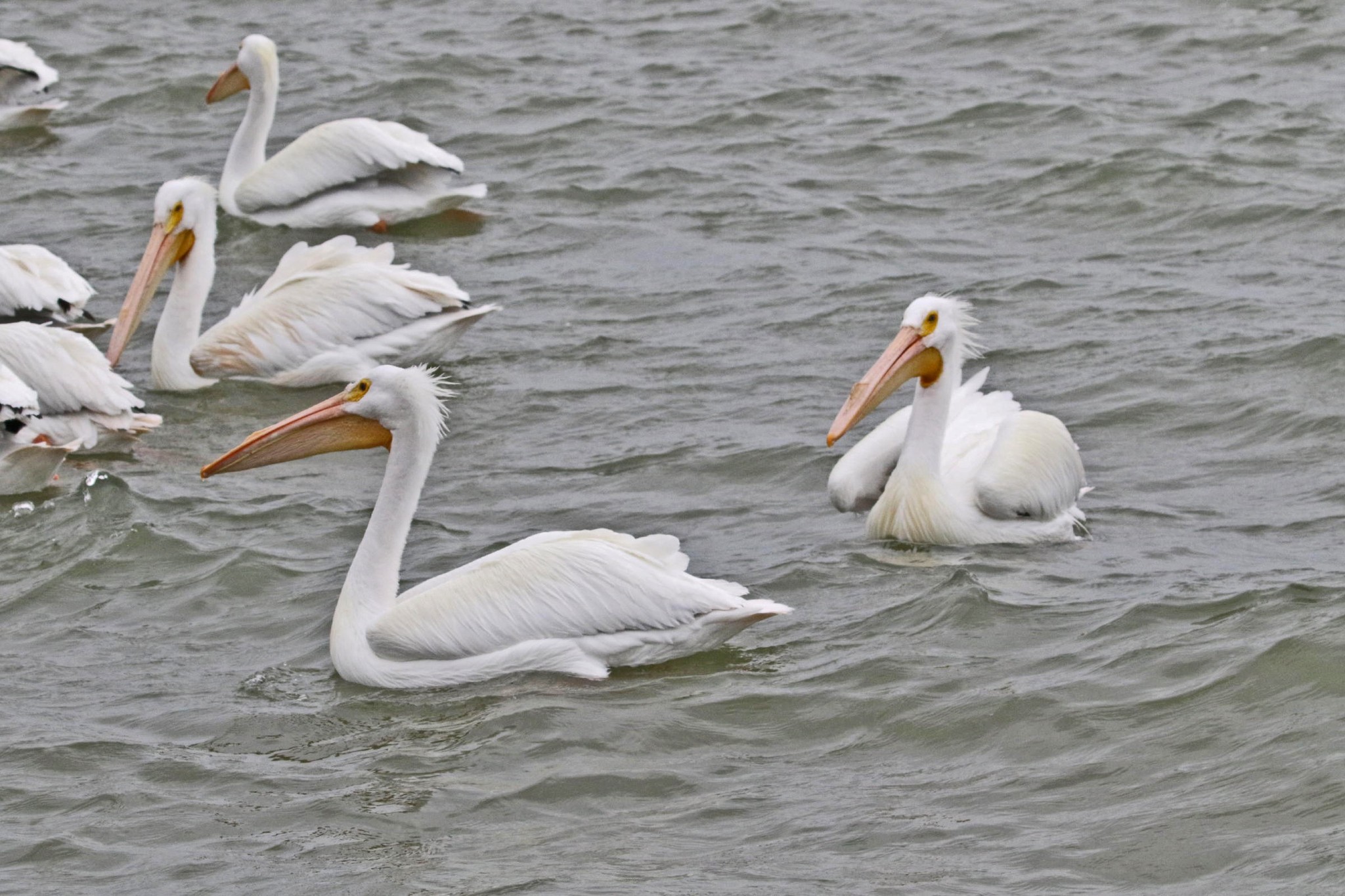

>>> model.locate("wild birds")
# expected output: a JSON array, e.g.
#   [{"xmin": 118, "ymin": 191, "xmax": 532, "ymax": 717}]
[
  {"xmin": 106, "ymin": 173, "xmax": 504, "ymax": 395},
  {"xmin": 200, "ymin": 363, "xmax": 796, "ymax": 691},
  {"xmin": 0, "ymin": 37, "xmax": 163, "ymax": 496},
  {"xmin": 206, "ymin": 32, "xmax": 487, "ymax": 228},
  {"xmin": 827, "ymin": 292, "xmax": 1087, "ymax": 544}
]
